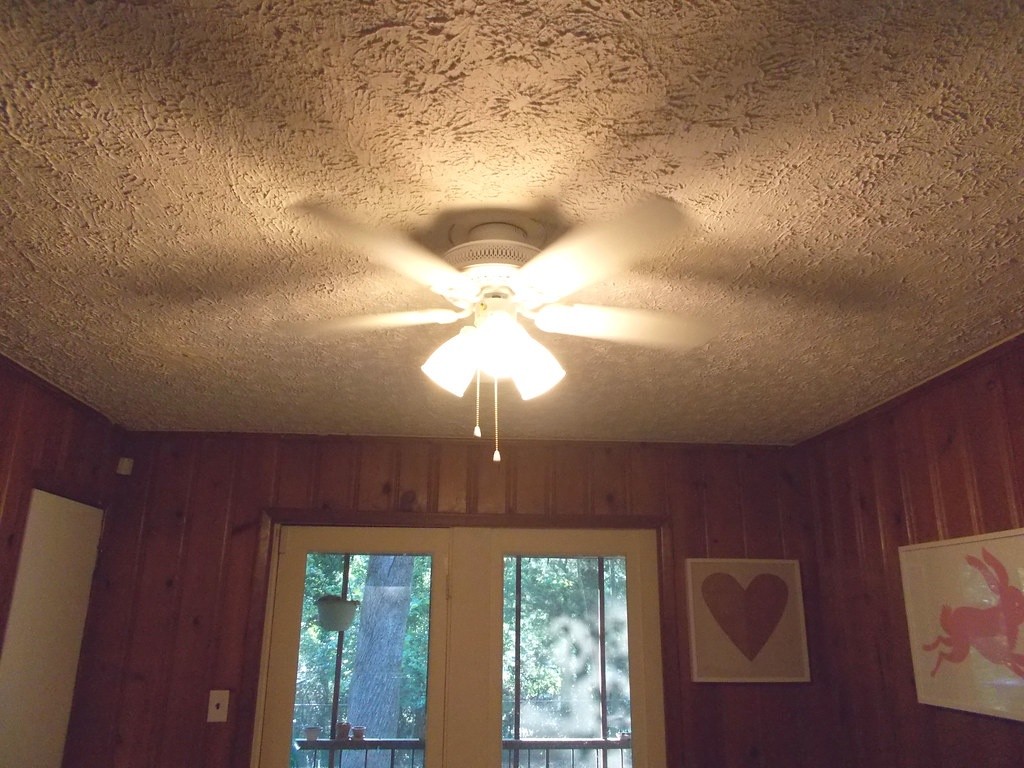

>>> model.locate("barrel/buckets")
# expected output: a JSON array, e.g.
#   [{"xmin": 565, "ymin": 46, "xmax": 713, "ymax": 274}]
[{"xmin": 316, "ymin": 579, "xmax": 360, "ymax": 630}]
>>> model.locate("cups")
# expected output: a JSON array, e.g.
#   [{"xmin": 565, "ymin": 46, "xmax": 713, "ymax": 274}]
[
  {"xmin": 351, "ymin": 726, "xmax": 366, "ymax": 740},
  {"xmin": 305, "ymin": 727, "xmax": 320, "ymax": 741},
  {"xmin": 336, "ymin": 722, "xmax": 351, "ymax": 739}
]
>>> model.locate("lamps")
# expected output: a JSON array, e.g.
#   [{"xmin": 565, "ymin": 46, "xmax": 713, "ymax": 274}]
[{"xmin": 428, "ymin": 313, "xmax": 567, "ymax": 466}]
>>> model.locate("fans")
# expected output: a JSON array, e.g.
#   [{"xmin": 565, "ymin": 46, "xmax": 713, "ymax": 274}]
[{"xmin": 265, "ymin": 180, "xmax": 718, "ymax": 394}]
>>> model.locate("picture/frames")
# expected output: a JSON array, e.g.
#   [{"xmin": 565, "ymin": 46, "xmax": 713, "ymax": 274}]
[
  {"xmin": 898, "ymin": 527, "xmax": 1024, "ymax": 720},
  {"xmin": 685, "ymin": 558, "xmax": 808, "ymax": 683}
]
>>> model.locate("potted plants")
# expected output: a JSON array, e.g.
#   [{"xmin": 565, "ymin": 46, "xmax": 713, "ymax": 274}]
[
  {"xmin": 335, "ymin": 716, "xmax": 350, "ymax": 738},
  {"xmin": 316, "ymin": 593, "xmax": 361, "ymax": 633}
]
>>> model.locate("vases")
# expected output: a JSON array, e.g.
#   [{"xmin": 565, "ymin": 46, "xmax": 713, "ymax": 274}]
[
  {"xmin": 304, "ymin": 726, "xmax": 320, "ymax": 740},
  {"xmin": 352, "ymin": 727, "xmax": 366, "ymax": 739}
]
